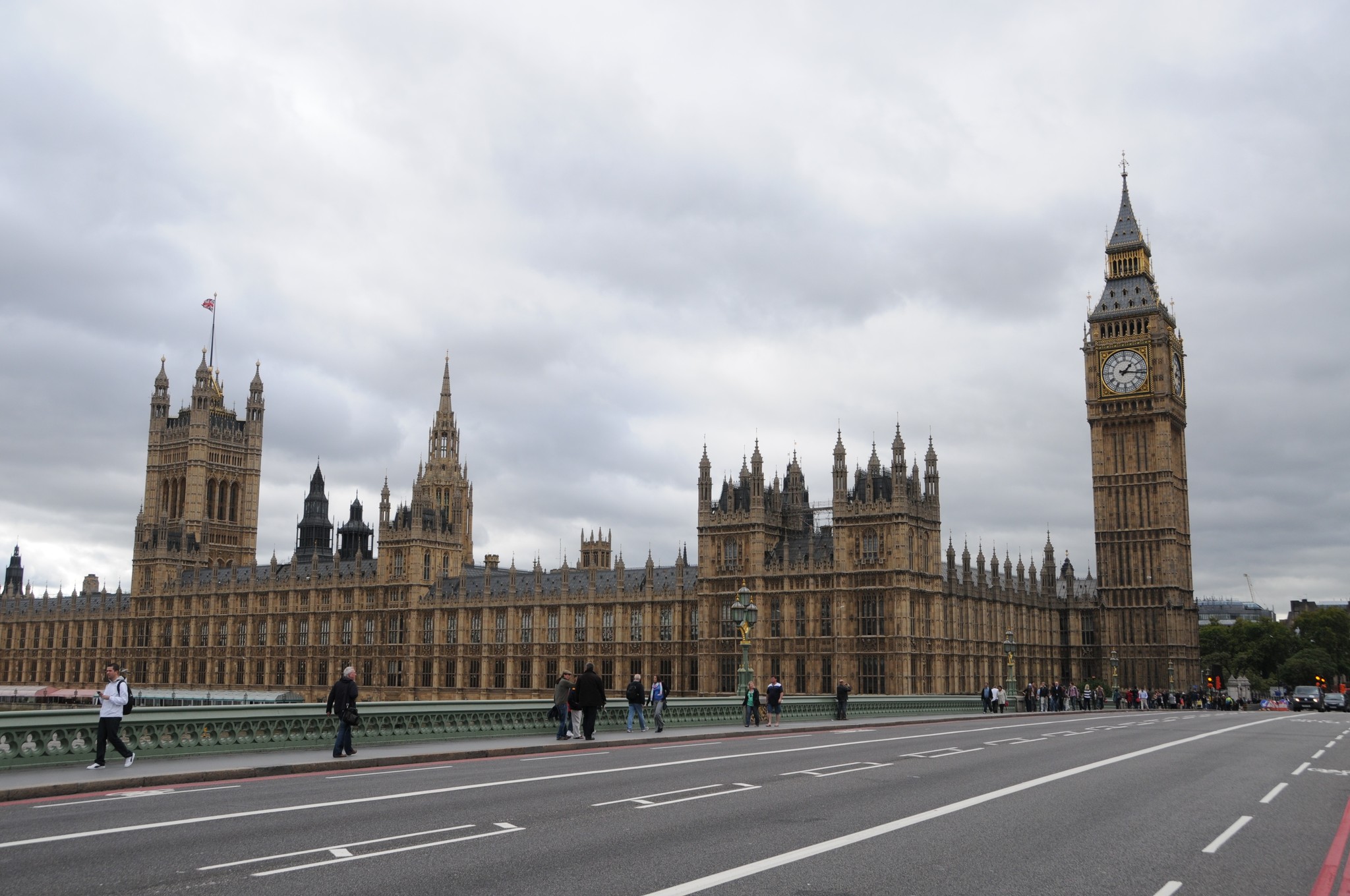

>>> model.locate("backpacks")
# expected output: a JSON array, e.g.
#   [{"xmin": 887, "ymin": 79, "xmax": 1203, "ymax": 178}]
[
  {"xmin": 626, "ymin": 681, "xmax": 640, "ymax": 700},
  {"xmin": 117, "ymin": 680, "xmax": 134, "ymax": 715}
]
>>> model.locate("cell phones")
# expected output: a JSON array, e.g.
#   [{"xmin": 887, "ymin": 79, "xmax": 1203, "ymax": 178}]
[
  {"xmin": 845, "ymin": 684, "xmax": 847, "ymax": 686},
  {"xmin": 97, "ymin": 691, "xmax": 101, "ymax": 694}
]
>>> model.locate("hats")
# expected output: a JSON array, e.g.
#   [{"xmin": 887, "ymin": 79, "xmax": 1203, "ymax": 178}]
[{"xmin": 563, "ymin": 670, "xmax": 572, "ymax": 674}]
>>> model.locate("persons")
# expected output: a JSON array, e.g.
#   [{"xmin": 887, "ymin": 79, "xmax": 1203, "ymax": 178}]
[
  {"xmin": 1112, "ymin": 685, "xmax": 1262, "ymax": 711},
  {"xmin": 554, "ymin": 670, "xmax": 583, "ymax": 741},
  {"xmin": 991, "ymin": 685, "xmax": 999, "ymax": 713},
  {"xmin": 626, "ymin": 674, "xmax": 649, "ymax": 732},
  {"xmin": 836, "ymin": 679, "xmax": 851, "ymax": 720},
  {"xmin": 741, "ymin": 681, "xmax": 761, "ymax": 728},
  {"xmin": 1022, "ymin": 681, "xmax": 1106, "ymax": 712},
  {"xmin": 645, "ymin": 674, "xmax": 670, "ymax": 733},
  {"xmin": 995, "ymin": 686, "xmax": 1007, "ymax": 713},
  {"xmin": 574, "ymin": 663, "xmax": 607, "ymax": 740},
  {"xmin": 765, "ymin": 675, "xmax": 784, "ymax": 727},
  {"xmin": 86, "ymin": 663, "xmax": 135, "ymax": 769},
  {"xmin": 326, "ymin": 666, "xmax": 358, "ymax": 758},
  {"xmin": 981, "ymin": 683, "xmax": 993, "ymax": 714}
]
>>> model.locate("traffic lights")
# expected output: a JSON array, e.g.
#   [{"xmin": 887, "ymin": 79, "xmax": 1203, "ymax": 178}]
[
  {"xmin": 1208, "ymin": 674, "xmax": 1212, "ymax": 688},
  {"xmin": 1316, "ymin": 674, "xmax": 1329, "ymax": 690}
]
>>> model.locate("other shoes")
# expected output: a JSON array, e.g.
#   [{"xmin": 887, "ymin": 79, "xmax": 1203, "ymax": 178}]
[
  {"xmin": 563, "ymin": 735, "xmax": 571, "ymax": 738},
  {"xmin": 838, "ymin": 718, "xmax": 840, "ymax": 720},
  {"xmin": 641, "ymin": 726, "xmax": 649, "ymax": 732},
  {"xmin": 556, "ymin": 736, "xmax": 568, "ymax": 740},
  {"xmin": 843, "ymin": 718, "xmax": 849, "ymax": 720},
  {"xmin": 627, "ymin": 730, "xmax": 634, "ymax": 732},
  {"xmin": 655, "ymin": 727, "xmax": 663, "ymax": 732},
  {"xmin": 586, "ymin": 737, "xmax": 595, "ymax": 740},
  {"xmin": 333, "ymin": 754, "xmax": 346, "ymax": 757},
  {"xmin": 347, "ymin": 750, "xmax": 357, "ymax": 755},
  {"xmin": 745, "ymin": 725, "xmax": 749, "ymax": 727},
  {"xmin": 573, "ymin": 736, "xmax": 584, "ymax": 739},
  {"xmin": 755, "ymin": 725, "xmax": 759, "ymax": 727},
  {"xmin": 592, "ymin": 729, "xmax": 596, "ymax": 734}
]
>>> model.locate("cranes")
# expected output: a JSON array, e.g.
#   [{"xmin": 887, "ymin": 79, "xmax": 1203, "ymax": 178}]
[{"xmin": 1244, "ymin": 573, "xmax": 1258, "ymax": 603}]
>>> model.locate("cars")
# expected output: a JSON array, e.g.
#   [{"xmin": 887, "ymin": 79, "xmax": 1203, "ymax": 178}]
[{"xmin": 1324, "ymin": 692, "xmax": 1350, "ymax": 713}]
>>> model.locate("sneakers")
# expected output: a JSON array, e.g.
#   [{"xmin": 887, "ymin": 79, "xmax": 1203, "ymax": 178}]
[
  {"xmin": 86, "ymin": 763, "xmax": 105, "ymax": 769},
  {"xmin": 124, "ymin": 752, "xmax": 135, "ymax": 767},
  {"xmin": 566, "ymin": 731, "xmax": 574, "ymax": 736}
]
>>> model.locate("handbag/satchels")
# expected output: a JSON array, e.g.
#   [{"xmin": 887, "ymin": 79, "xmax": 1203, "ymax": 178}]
[
  {"xmin": 1116, "ymin": 694, "xmax": 1121, "ymax": 699},
  {"xmin": 663, "ymin": 701, "xmax": 667, "ymax": 710},
  {"xmin": 1004, "ymin": 701, "xmax": 1009, "ymax": 707},
  {"xmin": 1124, "ymin": 694, "xmax": 1128, "ymax": 699},
  {"xmin": 548, "ymin": 705, "xmax": 559, "ymax": 721},
  {"xmin": 342, "ymin": 707, "xmax": 360, "ymax": 726}
]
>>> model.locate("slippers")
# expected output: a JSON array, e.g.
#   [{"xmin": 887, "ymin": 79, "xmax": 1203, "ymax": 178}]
[
  {"xmin": 766, "ymin": 724, "xmax": 771, "ymax": 726},
  {"xmin": 774, "ymin": 724, "xmax": 779, "ymax": 726}
]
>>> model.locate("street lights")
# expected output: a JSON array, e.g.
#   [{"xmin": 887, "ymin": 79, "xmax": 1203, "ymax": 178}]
[
  {"xmin": 1110, "ymin": 647, "xmax": 1119, "ymax": 698},
  {"xmin": 1168, "ymin": 659, "xmax": 1176, "ymax": 696},
  {"xmin": 731, "ymin": 578, "xmax": 758, "ymax": 696},
  {"xmin": 1004, "ymin": 626, "xmax": 1018, "ymax": 696}
]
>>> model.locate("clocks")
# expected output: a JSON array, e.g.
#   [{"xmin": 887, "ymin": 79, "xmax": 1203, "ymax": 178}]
[
  {"xmin": 1099, "ymin": 345, "xmax": 1151, "ymax": 398},
  {"xmin": 1173, "ymin": 350, "xmax": 1184, "ymax": 401}
]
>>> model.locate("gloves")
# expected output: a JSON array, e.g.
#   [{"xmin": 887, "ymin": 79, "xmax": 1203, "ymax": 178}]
[{"xmin": 327, "ymin": 712, "xmax": 331, "ymax": 717}]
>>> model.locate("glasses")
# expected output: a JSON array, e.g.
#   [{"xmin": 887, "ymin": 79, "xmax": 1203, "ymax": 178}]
[{"xmin": 350, "ymin": 672, "xmax": 357, "ymax": 674}]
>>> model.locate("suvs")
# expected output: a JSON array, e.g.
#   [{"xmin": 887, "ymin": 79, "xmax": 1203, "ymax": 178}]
[{"xmin": 1290, "ymin": 686, "xmax": 1325, "ymax": 713}]
